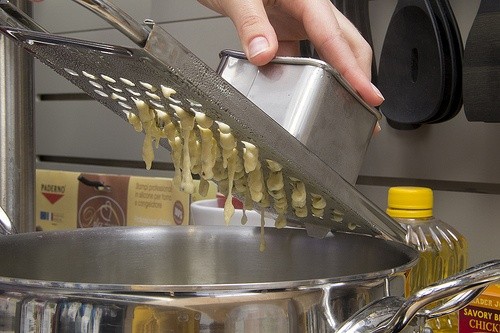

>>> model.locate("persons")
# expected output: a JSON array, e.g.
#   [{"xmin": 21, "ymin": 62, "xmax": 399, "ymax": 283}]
[{"xmin": 199, "ymin": 0, "xmax": 388, "ymax": 136}]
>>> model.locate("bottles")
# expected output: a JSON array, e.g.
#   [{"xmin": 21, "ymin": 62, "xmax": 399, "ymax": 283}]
[{"xmin": 384, "ymin": 185, "xmax": 467, "ymax": 332}]
